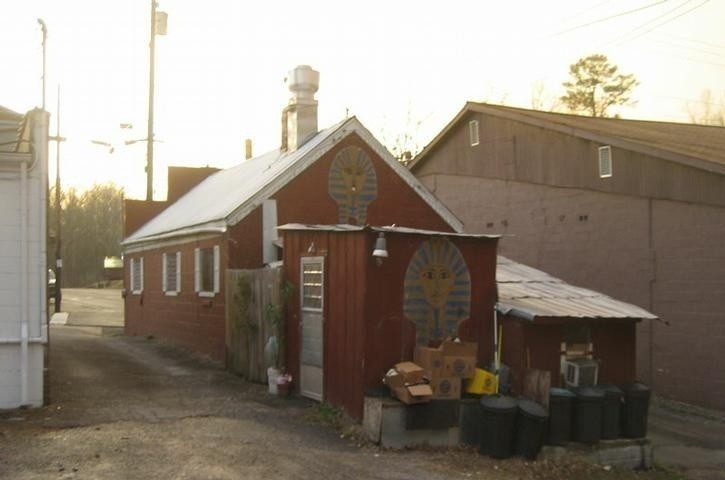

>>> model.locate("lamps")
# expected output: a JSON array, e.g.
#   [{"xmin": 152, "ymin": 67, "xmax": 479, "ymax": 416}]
[{"xmin": 373, "ymin": 235, "xmax": 389, "ymax": 259}]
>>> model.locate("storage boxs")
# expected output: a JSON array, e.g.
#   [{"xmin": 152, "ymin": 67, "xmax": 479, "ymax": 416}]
[{"xmin": 384, "ymin": 336, "xmax": 511, "ymax": 405}]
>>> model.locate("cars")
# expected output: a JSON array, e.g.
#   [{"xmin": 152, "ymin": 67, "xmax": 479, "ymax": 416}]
[{"xmin": 48, "ymin": 269, "xmax": 61, "ymax": 302}]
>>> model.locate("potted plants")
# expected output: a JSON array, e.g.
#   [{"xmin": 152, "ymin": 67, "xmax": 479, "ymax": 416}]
[{"xmin": 262, "ymin": 277, "xmax": 293, "ymax": 396}]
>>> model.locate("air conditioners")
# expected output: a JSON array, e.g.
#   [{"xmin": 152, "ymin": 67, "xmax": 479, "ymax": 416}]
[{"xmin": 566, "ymin": 358, "xmax": 599, "ymax": 386}]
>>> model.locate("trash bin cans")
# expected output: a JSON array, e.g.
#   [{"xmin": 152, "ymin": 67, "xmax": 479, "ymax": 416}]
[{"xmin": 457, "ymin": 383, "xmax": 652, "ymax": 460}]
